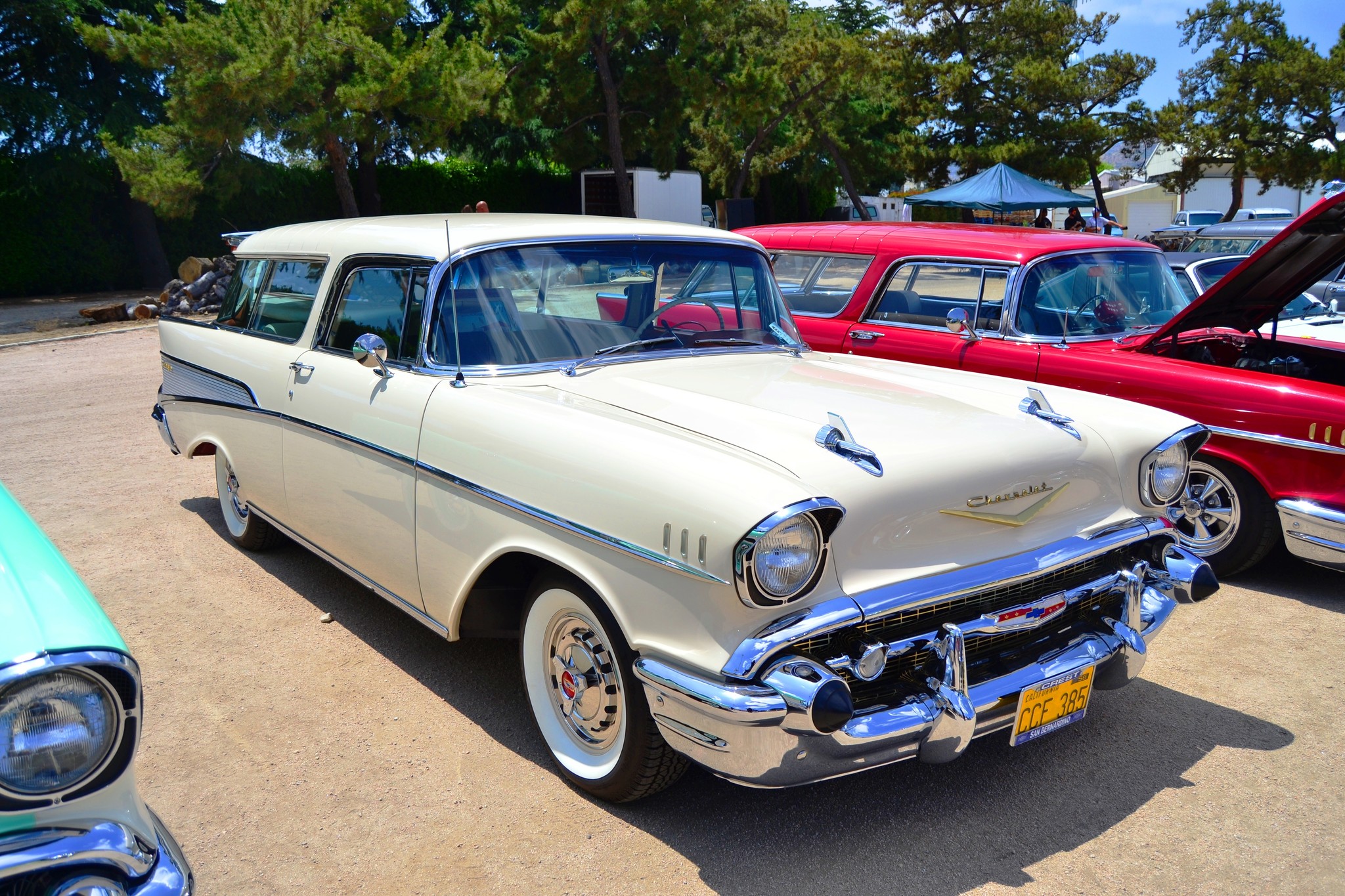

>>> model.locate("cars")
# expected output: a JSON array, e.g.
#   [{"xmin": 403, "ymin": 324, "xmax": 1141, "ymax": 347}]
[
  {"xmin": 1, "ymin": 481, "xmax": 198, "ymax": 896},
  {"xmin": 595, "ymin": 179, "xmax": 1345, "ymax": 582},
  {"xmin": 150, "ymin": 201, "xmax": 1232, "ymax": 809}
]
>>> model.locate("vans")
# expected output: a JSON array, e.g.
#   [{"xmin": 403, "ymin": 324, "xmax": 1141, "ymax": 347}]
[{"xmin": 1232, "ymin": 206, "xmax": 1295, "ymax": 222}]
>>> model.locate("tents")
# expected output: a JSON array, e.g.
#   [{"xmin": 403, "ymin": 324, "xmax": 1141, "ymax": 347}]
[{"xmin": 903, "ymin": 162, "xmax": 1097, "ymax": 233}]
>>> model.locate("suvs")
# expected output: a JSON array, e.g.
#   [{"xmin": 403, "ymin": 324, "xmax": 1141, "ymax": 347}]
[{"xmin": 1170, "ymin": 208, "xmax": 1227, "ymax": 228}]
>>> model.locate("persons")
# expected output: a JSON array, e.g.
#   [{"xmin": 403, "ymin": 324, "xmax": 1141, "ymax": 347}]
[
  {"xmin": 1034, "ymin": 207, "xmax": 1052, "ymax": 229},
  {"xmin": 1064, "ymin": 208, "xmax": 1086, "ymax": 232},
  {"xmin": 461, "ymin": 204, "xmax": 472, "ymax": 213},
  {"xmin": 1087, "ymin": 206, "xmax": 1125, "ymax": 234},
  {"xmin": 1136, "ymin": 229, "xmax": 1268, "ymax": 258},
  {"xmin": 476, "ymin": 201, "xmax": 489, "ymax": 212}
]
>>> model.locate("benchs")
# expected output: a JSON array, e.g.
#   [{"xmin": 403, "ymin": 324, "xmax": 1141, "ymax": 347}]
[
  {"xmin": 443, "ymin": 324, "xmax": 645, "ymax": 366},
  {"xmin": 755, "ymin": 287, "xmax": 927, "ymax": 316}
]
